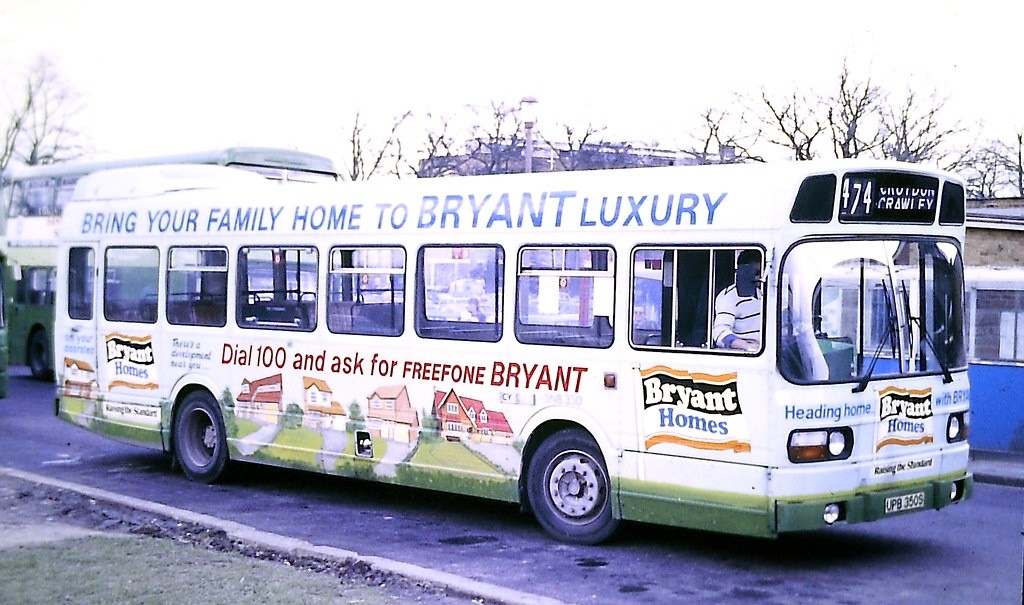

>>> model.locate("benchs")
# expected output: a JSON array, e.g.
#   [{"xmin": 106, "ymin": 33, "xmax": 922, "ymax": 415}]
[{"xmin": 76, "ymin": 292, "xmax": 366, "ymax": 332}]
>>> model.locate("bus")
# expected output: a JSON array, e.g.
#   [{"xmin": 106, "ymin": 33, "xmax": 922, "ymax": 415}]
[
  {"xmin": 1, "ymin": 145, "xmax": 355, "ymax": 383},
  {"xmin": 53, "ymin": 158, "xmax": 974, "ymax": 547}
]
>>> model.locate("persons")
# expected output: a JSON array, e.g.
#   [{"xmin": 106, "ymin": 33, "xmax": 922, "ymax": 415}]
[{"xmin": 712, "ymin": 251, "xmax": 762, "ymax": 352}]
